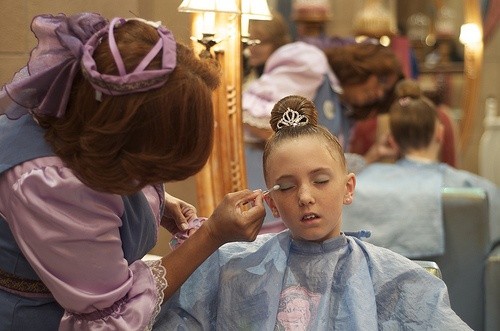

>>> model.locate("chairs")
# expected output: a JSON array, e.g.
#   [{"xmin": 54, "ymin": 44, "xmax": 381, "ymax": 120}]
[{"xmin": 423, "ymin": 188, "xmax": 500, "ymax": 331}]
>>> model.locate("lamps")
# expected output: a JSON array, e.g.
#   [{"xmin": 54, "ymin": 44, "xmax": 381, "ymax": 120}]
[
  {"xmin": 240, "ymin": 0, "xmax": 272, "ymax": 50},
  {"xmin": 177, "ymin": 0, "xmax": 240, "ymax": 66}
]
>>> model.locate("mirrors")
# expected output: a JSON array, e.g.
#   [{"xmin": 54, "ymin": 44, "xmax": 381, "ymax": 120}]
[{"xmin": 188, "ymin": 0, "xmax": 500, "ymax": 331}]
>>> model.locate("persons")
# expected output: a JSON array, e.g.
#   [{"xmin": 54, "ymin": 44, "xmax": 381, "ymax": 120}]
[
  {"xmin": 241, "ymin": 30, "xmax": 408, "ymax": 234},
  {"xmin": 147, "ymin": 94, "xmax": 475, "ymax": 331},
  {"xmin": 349, "ymin": 81, "xmax": 500, "ymax": 261},
  {"xmin": 0, "ymin": 11, "xmax": 268, "ymax": 331}
]
